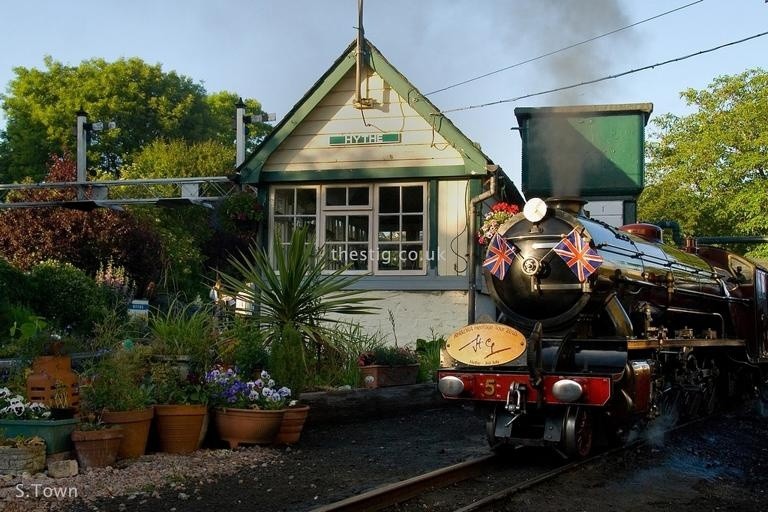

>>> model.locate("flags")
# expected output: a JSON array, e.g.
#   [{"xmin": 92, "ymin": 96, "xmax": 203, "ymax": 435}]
[
  {"xmin": 481, "ymin": 233, "xmax": 519, "ymax": 282},
  {"xmin": 554, "ymin": 230, "xmax": 603, "ymax": 282}
]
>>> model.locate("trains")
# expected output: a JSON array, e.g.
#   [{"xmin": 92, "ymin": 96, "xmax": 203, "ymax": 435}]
[{"xmin": 435, "ymin": 102, "xmax": 768, "ymax": 462}]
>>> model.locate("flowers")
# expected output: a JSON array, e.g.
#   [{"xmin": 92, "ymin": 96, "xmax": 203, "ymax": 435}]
[{"xmin": 476, "ymin": 202, "xmax": 518, "ymax": 244}]
